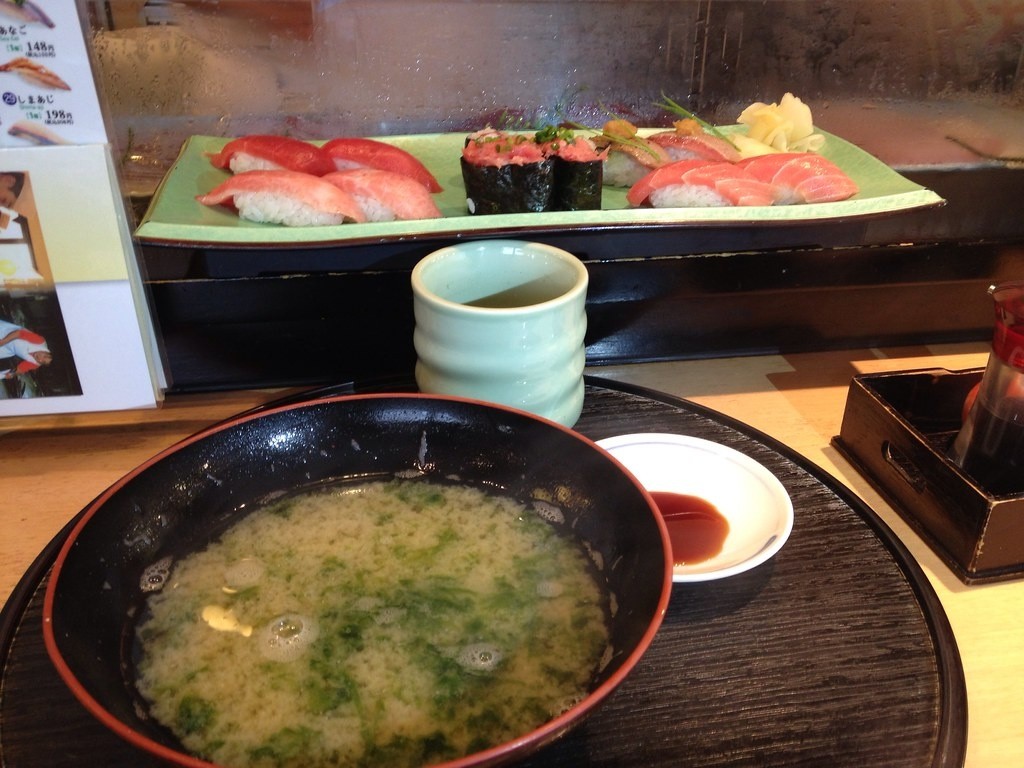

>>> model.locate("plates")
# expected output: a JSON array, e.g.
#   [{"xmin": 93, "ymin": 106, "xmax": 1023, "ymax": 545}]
[
  {"xmin": 130, "ymin": 112, "xmax": 942, "ymax": 255},
  {"xmin": 588, "ymin": 431, "xmax": 795, "ymax": 587}
]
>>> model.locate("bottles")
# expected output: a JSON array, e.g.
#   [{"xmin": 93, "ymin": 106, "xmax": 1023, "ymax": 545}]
[{"xmin": 938, "ymin": 279, "xmax": 1024, "ymax": 494}]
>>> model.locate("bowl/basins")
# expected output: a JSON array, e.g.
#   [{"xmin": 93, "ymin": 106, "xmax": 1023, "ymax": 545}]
[{"xmin": 38, "ymin": 390, "xmax": 675, "ymax": 768}]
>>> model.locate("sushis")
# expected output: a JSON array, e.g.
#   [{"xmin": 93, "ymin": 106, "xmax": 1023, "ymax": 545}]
[{"xmin": 194, "ymin": 92, "xmax": 859, "ymax": 226}]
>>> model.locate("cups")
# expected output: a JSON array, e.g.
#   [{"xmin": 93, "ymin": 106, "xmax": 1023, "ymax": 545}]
[{"xmin": 413, "ymin": 239, "xmax": 592, "ymax": 432}]
